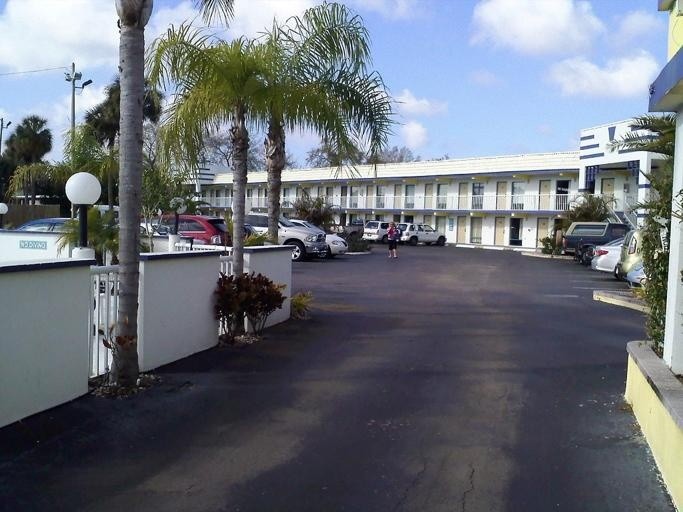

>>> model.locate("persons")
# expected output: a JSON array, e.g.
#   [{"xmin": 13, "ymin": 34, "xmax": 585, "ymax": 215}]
[{"xmin": 385, "ymin": 221, "xmax": 398, "ymax": 258}]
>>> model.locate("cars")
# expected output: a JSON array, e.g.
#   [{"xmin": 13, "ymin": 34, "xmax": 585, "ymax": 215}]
[
  {"xmin": 619, "ymin": 227, "xmax": 648, "ymax": 286},
  {"xmin": 14, "ymin": 217, "xmax": 77, "ymax": 232},
  {"xmin": 592, "ymin": 239, "xmax": 623, "ymax": 279}
]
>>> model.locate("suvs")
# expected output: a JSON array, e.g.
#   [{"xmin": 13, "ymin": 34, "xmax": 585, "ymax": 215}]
[
  {"xmin": 361, "ymin": 221, "xmax": 390, "ymax": 242},
  {"xmin": 397, "ymin": 223, "xmax": 447, "ymax": 246},
  {"xmin": 140, "ymin": 214, "xmax": 234, "ymax": 248},
  {"xmin": 561, "ymin": 220, "xmax": 629, "ymax": 263},
  {"xmin": 244, "ymin": 213, "xmax": 348, "ymax": 261}
]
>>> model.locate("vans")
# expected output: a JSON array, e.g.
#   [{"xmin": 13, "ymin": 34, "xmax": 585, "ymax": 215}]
[{"xmin": 76, "ymin": 204, "xmax": 119, "ymax": 223}]
